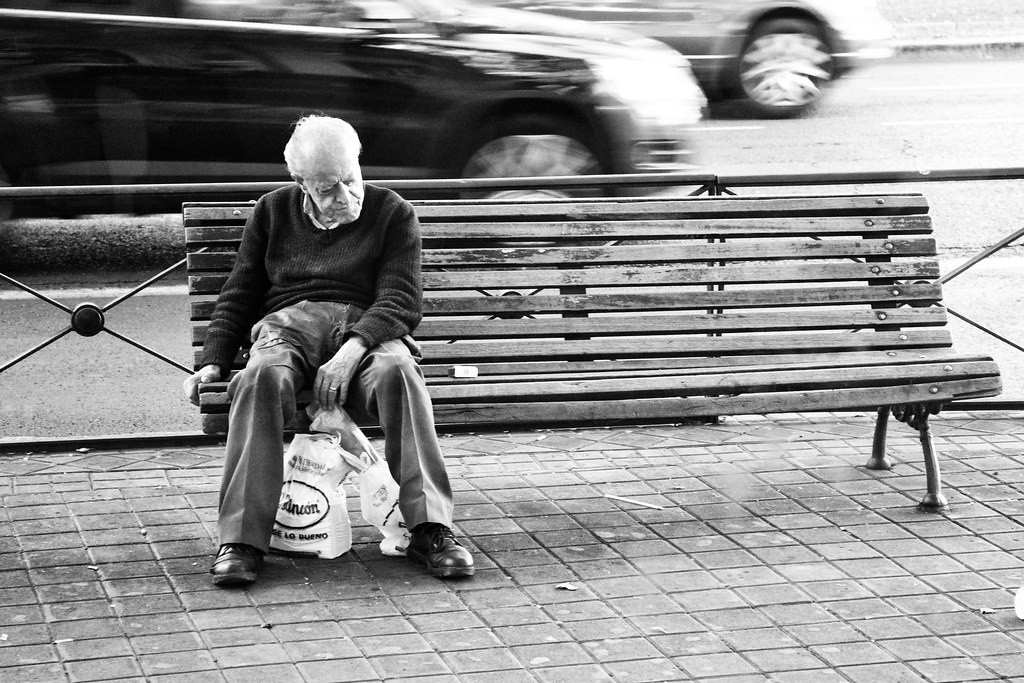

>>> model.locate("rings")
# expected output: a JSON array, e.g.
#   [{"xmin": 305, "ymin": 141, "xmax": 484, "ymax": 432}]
[{"xmin": 329, "ymin": 387, "xmax": 338, "ymax": 391}]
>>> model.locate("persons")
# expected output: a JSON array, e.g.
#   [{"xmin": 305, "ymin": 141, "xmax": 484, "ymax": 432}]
[{"xmin": 183, "ymin": 112, "xmax": 475, "ymax": 587}]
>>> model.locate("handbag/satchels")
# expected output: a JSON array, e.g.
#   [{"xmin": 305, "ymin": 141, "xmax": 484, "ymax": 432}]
[{"xmin": 269, "ymin": 399, "xmax": 412, "ymax": 560}]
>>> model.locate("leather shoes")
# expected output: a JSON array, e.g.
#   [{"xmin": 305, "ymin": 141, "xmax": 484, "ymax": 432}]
[
  {"xmin": 407, "ymin": 523, "xmax": 475, "ymax": 577},
  {"xmin": 212, "ymin": 542, "xmax": 264, "ymax": 583}
]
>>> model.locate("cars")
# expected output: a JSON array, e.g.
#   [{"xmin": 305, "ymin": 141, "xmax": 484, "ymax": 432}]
[
  {"xmin": 1, "ymin": 0, "xmax": 709, "ymax": 266},
  {"xmin": 475, "ymin": 0, "xmax": 899, "ymax": 119}
]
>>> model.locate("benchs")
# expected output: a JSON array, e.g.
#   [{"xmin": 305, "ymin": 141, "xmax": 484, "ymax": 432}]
[{"xmin": 182, "ymin": 191, "xmax": 1004, "ymax": 515}]
